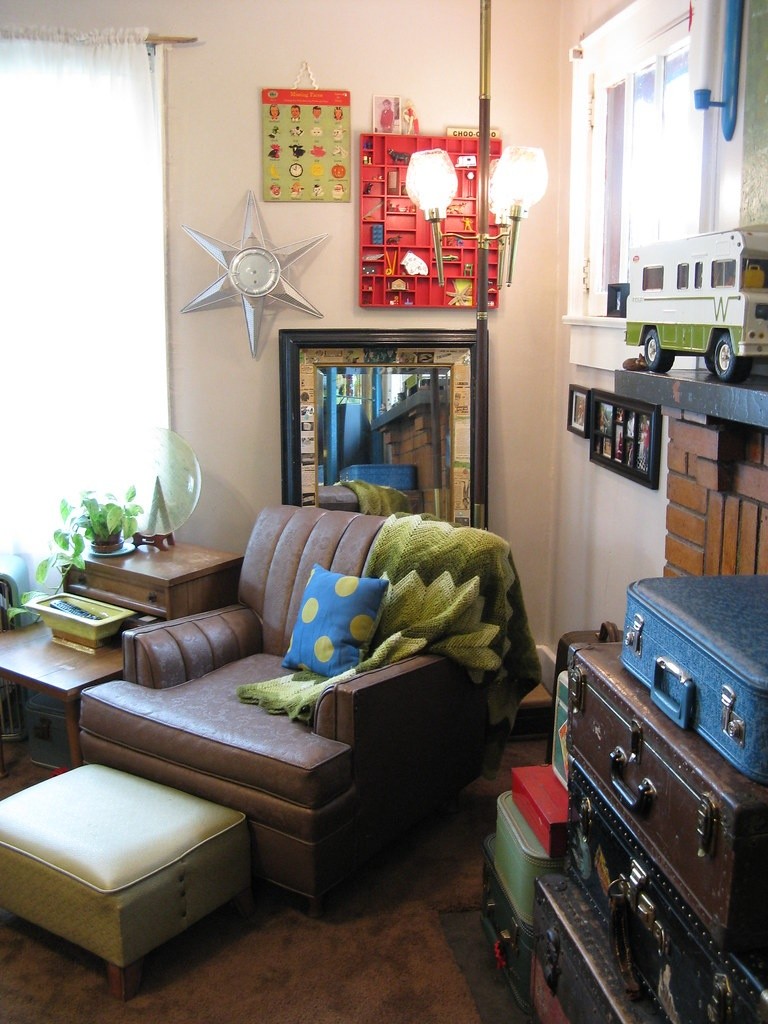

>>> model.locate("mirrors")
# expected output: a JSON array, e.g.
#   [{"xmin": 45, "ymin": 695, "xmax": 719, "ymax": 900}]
[{"xmin": 279, "ymin": 328, "xmax": 489, "ymax": 531}]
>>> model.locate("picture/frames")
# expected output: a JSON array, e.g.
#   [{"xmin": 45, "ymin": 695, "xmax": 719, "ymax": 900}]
[
  {"xmin": 567, "ymin": 383, "xmax": 589, "ymax": 438},
  {"xmin": 589, "ymin": 388, "xmax": 662, "ymax": 490},
  {"xmin": 373, "ymin": 94, "xmax": 402, "ymax": 135}
]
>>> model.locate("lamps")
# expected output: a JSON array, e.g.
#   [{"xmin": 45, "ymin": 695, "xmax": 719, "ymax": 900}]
[{"xmin": 405, "ymin": 0, "xmax": 550, "ymax": 288}]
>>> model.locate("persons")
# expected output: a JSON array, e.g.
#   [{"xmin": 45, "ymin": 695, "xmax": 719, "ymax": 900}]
[
  {"xmin": 381, "ymin": 100, "xmax": 394, "ymax": 133},
  {"xmin": 461, "ymin": 217, "xmax": 474, "ymax": 232},
  {"xmin": 403, "ymin": 98, "xmax": 417, "ymax": 135},
  {"xmin": 577, "ymin": 396, "xmax": 652, "ymax": 475}
]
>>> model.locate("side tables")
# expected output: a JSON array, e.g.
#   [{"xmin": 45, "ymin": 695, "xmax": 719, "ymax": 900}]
[{"xmin": 0, "ymin": 621, "xmax": 123, "ymax": 769}]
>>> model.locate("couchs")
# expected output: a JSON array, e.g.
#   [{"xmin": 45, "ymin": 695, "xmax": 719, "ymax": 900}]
[
  {"xmin": 79, "ymin": 505, "xmax": 541, "ymax": 919},
  {"xmin": 312, "ymin": 483, "xmax": 427, "ymax": 517}
]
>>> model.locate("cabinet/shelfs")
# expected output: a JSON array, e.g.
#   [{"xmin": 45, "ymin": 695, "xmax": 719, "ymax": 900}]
[
  {"xmin": 358, "ymin": 132, "xmax": 502, "ymax": 310},
  {"xmin": 63, "ymin": 542, "xmax": 244, "ymax": 638}
]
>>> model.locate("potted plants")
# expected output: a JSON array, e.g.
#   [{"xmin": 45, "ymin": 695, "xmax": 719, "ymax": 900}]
[{"xmin": 7, "ymin": 485, "xmax": 144, "ymax": 628}]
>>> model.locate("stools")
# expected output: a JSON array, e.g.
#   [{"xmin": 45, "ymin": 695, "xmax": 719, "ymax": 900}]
[{"xmin": 0, "ymin": 763, "xmax": 252, "ymax": 1002}]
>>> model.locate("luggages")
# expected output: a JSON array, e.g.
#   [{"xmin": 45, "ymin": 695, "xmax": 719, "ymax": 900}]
[{"xmin": 545, "ymin": 622, "xmax": 625, "ymax": 763}]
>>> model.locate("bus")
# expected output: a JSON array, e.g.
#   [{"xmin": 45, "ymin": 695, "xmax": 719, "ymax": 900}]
[{"xmin": 624, "ymin": 222, "xmax": 768, "ymax": 386}]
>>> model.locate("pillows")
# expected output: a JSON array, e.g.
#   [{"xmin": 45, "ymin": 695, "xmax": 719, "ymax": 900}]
[{"xmin": 281, "ymin": 563, "xmax": 390, "ymax": 678}]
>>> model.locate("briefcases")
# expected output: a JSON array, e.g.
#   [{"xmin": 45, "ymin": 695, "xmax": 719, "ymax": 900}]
[
  {"xmin": 339, "ymin": 465, "xmax": 416, "ymax": 490},
  {"xmin": 567, "ymin": 642, "xmax": 768, "ymax": 948},
  {"xmin": 565, "ymin": 759, "xmax": 768, "ymax": 1024},
  {"xmin": 619, "ymin": 576, "xmax": 768, "ymax": 784},
  {"xmin": 533, "ymin": 874, "xmax": 671, "ymax": 1024},
  {"xmin": 480, "ymin": 834, "xmax": 534, "ymax": 1015}
]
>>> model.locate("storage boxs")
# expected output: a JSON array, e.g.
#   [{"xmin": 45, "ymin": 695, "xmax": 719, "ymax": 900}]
[
  {"xmin": 494, "ymin": 790, "xmax": 561, "ymax": 925},
  {"xmin": 25, "ymin": 676, "xmax": 122, "ymax": 771},
  {"xmin": 550, "ymin": 670, "xmax": 568, "ymax": 790},
  {"xmin": 511, "ymin": 764, "xmax": 571, "ymax": 857}
]
